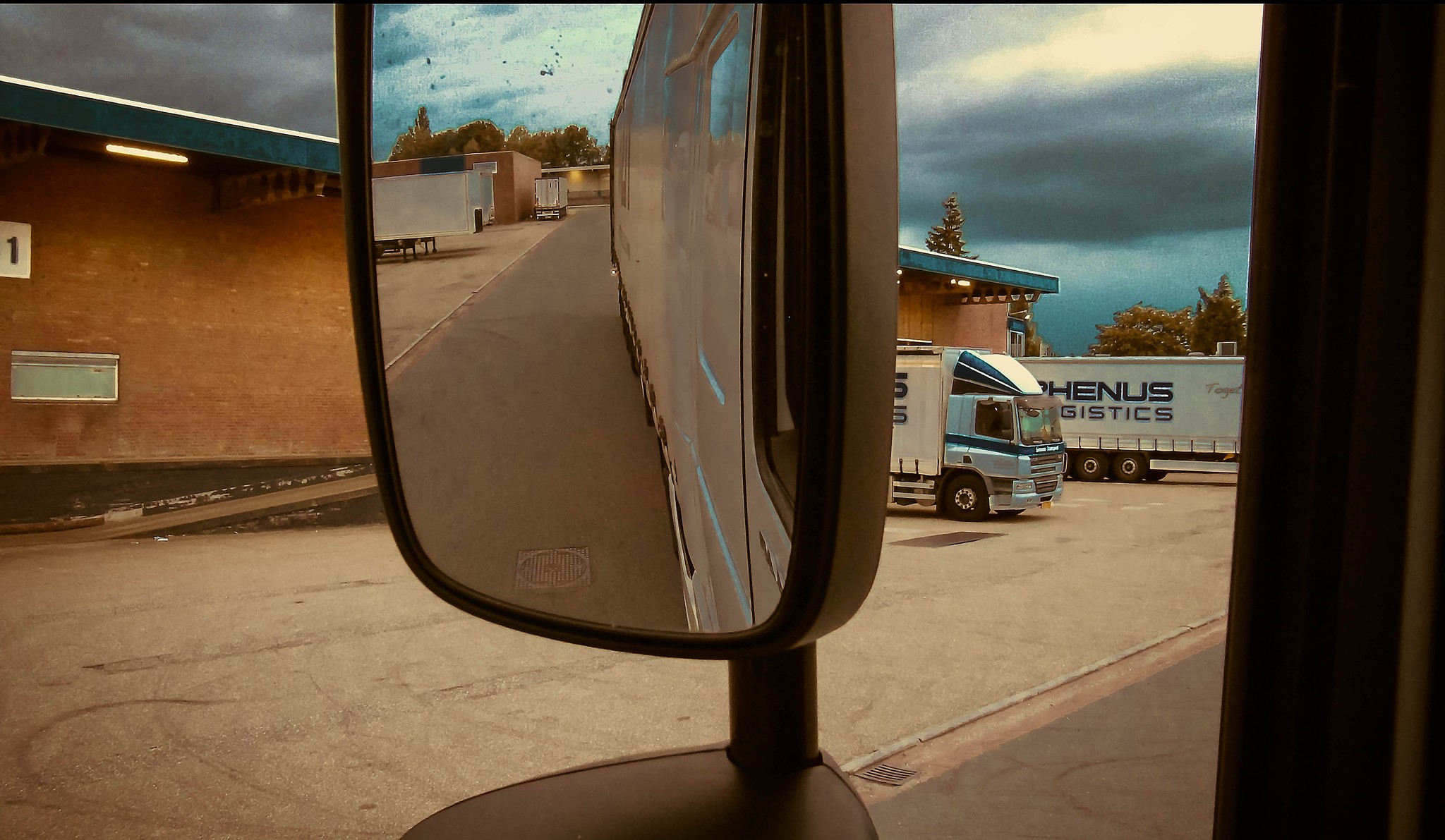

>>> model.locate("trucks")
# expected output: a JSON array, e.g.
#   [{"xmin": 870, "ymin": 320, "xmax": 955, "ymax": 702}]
[{"xmin": 888, "ymin": 338, "xmax": 1070, "ymax": 524}]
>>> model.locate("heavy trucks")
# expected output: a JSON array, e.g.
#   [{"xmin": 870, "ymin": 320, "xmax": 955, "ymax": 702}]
[
  {"xmin": 1012, "ymin": 340, "xmax": 1245, "ymax": 485},
  {"xmin": 533, "ymin": 176, "xmax": 571, "ymax": 221}
]
